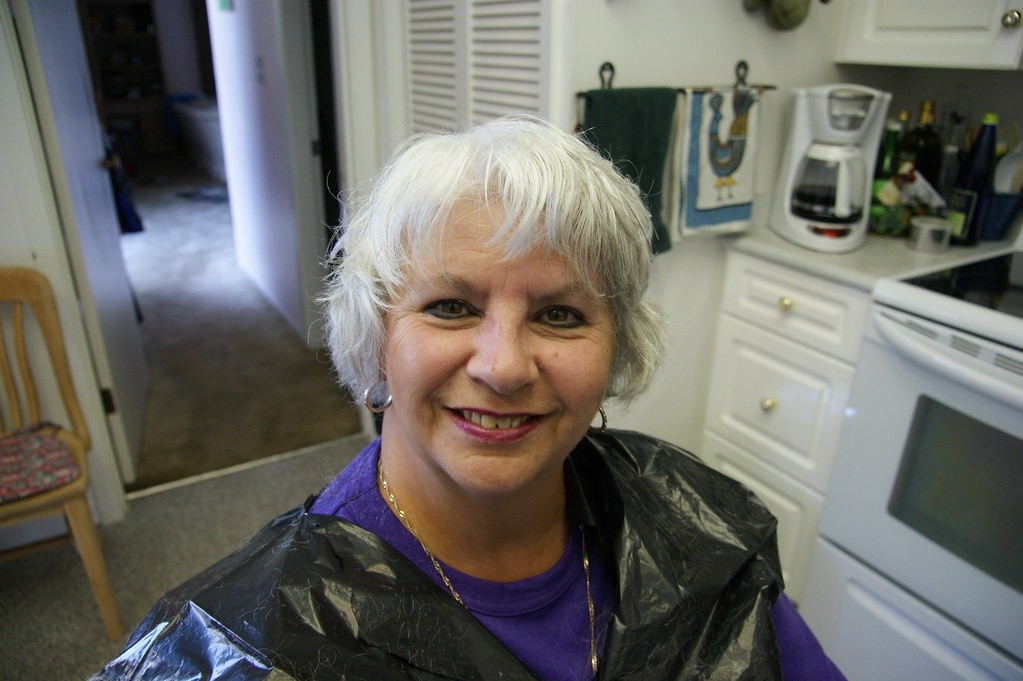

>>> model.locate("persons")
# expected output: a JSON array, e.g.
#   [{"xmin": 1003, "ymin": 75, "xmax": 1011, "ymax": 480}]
[{"xmin": 82, "ymin": 112, "xmax": 846, "ymax": 681}]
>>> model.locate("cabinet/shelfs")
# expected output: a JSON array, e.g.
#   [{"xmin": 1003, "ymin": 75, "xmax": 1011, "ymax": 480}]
[
  {"xmin": 102, "ymin": 95, "xmax": 176, "ymax": 155},
  {"xmin": 77, "ymin": 0, "xmax": 165, "ymax": 99},
  {"xmin": 832, "ymin": 0, "xmax": 1023, "ymax": 72},
  {"xmin": 700, "ymin": 240, "xmax": 872, "ymax": 609}
]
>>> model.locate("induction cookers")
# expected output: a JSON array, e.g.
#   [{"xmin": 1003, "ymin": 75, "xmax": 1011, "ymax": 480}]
[{"xmin": 866, "ymin": 226, "xmax": 1023, "ymax": 349}]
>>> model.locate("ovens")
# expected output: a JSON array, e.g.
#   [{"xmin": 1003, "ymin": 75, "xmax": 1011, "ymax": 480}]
[{"xmin": 796, "ymin": 300, "xmax": 1023, "ymax": 681}]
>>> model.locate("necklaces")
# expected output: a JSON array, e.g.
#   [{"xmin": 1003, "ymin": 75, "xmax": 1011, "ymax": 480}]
[{"xmin": 381, "ymin": 480, "xmax": 599, "ymax": 678}]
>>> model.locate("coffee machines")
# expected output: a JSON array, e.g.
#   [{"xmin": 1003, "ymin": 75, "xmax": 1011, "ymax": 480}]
[{"xmin": 766, "ymin": 83, "xmax": 893, "ymax": 253}]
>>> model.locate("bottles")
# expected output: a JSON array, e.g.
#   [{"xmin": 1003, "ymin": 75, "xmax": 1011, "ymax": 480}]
[{"xmin": 869, "ymin": 100, "xmax": 1008, "ymax": 248}]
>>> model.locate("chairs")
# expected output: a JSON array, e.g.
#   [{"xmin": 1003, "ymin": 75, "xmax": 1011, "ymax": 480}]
[{"xmin": 0, "ymin": 266, "xmax": 127, "ymax": 642}]
